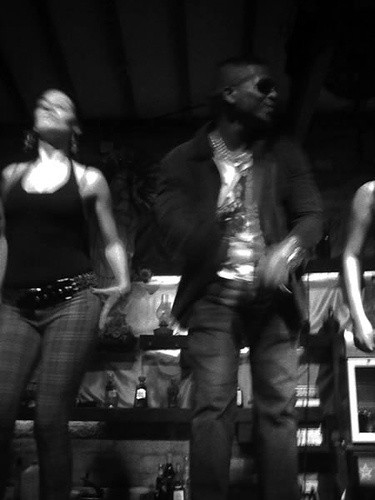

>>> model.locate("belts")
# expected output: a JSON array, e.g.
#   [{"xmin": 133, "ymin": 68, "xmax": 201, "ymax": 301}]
[{"xmin": 15, "ymin": 274, "xmax": 96, "ymax": 306}]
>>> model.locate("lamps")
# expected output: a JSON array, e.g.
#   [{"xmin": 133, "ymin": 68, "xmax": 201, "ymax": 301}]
[{"xmin": 153, "ymin": 293, "xmax": 172, "ymax": 334}]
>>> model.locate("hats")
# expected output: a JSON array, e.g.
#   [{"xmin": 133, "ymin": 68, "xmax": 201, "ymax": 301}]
[{"xmin": 207, "ymin": 55, "xmax": 266, "ymax": 99}]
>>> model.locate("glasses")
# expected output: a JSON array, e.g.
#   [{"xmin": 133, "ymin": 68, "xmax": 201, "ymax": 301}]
[{"xmin": 232, "ymin": 79, "xmax": 278, "ymax": 95}]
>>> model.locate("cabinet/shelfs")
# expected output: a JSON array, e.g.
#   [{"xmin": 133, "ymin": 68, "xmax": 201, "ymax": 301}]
[{"xmin": 16, "ymin": 332, "xmax": 350, "ymax": 500}]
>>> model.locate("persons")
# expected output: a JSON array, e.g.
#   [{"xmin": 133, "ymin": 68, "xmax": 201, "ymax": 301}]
[
  {"xmin": 0, "ymin": 88, "xmax": 131, "ymax": 500},
  {"xmin": 151, "ymin": 55, "xmax": 324, "ymax": 500},
  {"xmin": 341, "ymin": 180, "xmax": 375, "ymax": 351}
]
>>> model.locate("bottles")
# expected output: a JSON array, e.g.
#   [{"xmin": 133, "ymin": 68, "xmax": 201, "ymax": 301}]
[
  {"xmin": 133, "ymin": 377, "xmax": 148, "ymax": 408},
  {"xmin": 20, "ymin": 461, "xmax": 39, "ymax": 500},
  {"xmin": 154, "ymin": 454, "xmax": 192, "ymax": 500},
  {"xmin": 105, "ymin": 373, "xmax": 118, "ymax": 407},
  {"xmin": 359, "ymin": 410, "xmax": 374, "ymax": 432},
  {"xmin": 167, "ymin": 378, "xmax": 180, "ymax": 408},
  {"xmin": 236, "ymin": 387, "xmax": 242, "ymax": 408}
]
